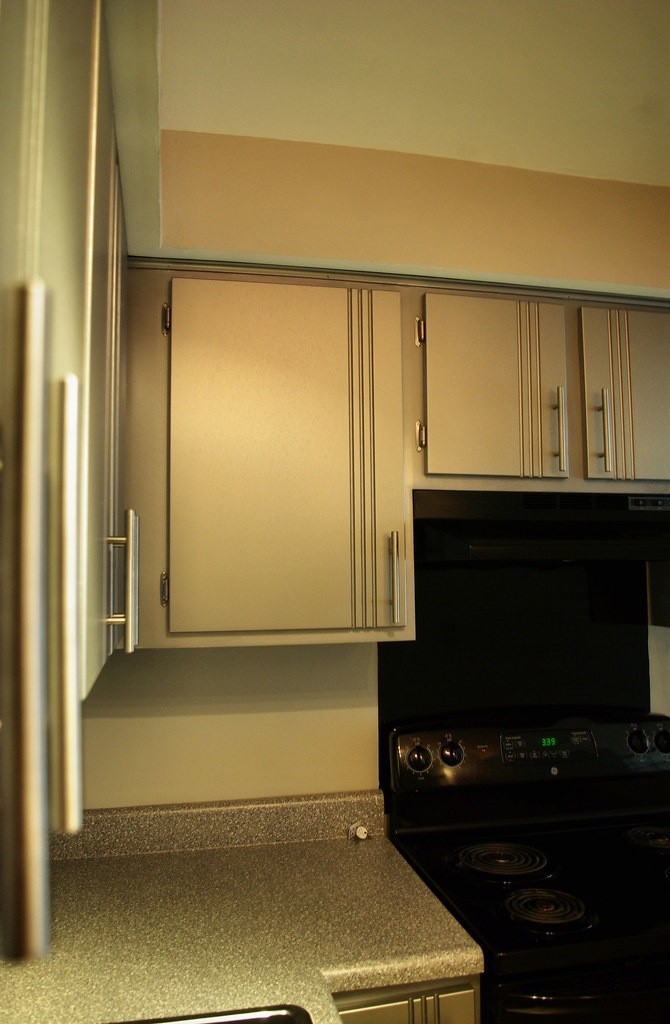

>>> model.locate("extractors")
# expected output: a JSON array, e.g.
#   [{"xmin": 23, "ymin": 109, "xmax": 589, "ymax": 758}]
[{"xmin": 415, "ymin": 491, "xmax": 670, "ymax": 570}]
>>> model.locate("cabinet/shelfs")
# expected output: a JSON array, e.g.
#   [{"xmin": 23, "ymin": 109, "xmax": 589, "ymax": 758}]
[
  {"xmin": 125, "ymin": 268, "xmax": 416, "ymax": 649},
  {"xmin": 414, "ymin": 286, "xmax": 670, "ymax": 494},
  {"xmin": 0, "ymin": 0, "xmax": 126, "ymax": 965}
]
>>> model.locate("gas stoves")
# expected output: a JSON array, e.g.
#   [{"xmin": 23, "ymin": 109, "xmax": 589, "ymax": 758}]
[{"xmin": 380, "ymin": 705, "xmax": 670, "ymax": 971}]
